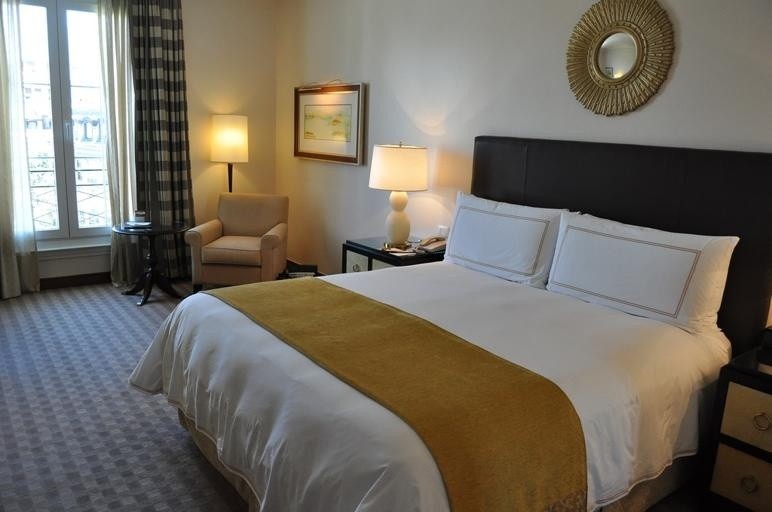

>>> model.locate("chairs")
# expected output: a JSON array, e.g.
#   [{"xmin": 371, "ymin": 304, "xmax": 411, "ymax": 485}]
[{"xmin": 184, "ymin": 193, "xmax": 292, "ymax": 288}]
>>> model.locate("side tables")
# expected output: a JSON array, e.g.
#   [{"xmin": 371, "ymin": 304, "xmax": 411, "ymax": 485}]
[{"xmin": 109, "ymin": 218, "xmax": 192, "ymax": 307}]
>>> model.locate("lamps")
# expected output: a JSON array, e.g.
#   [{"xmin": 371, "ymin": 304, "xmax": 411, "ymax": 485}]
[
  {"xmin": 209, "ymin": 113, "xmax": 251, "ymax": 192},
  {"xmin": 367, "ymin": 140, "xmax": 432, "ymax": 249}
]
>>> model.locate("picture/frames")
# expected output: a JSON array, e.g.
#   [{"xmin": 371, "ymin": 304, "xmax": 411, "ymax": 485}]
[
  {"xmin": 604, "ymin": 66, "xmax": 614, "ymax": 77},
  {"xmin": 292, "ymin": 81, "xmax": 365, "ymax": 167}
]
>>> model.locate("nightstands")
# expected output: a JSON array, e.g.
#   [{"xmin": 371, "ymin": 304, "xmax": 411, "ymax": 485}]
[
  {"xmin": 341, "ymin": 235, "xmax": 446, "ymax": 275},
  {"xmin": 693, "ymin": 342, "xmax": 771, "ymax": 512}
]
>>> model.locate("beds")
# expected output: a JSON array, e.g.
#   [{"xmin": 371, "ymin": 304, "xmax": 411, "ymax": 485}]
[{"xmin": 127, "ymin": 133, "xmax": 771, "ymax": 512}]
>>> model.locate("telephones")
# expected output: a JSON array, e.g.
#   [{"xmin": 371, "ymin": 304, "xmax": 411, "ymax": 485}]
[{"xmin": 413, "ymin": 235, "xmax": 447, "ymax": 253}]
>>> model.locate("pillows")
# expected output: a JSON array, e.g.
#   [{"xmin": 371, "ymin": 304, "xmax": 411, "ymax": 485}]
[
  {"xmin": 445, "ymin": 189, "xmax": 565, "ymax": 294},
  {"xmin": 545, "ymin": 209, "xmax": 744, "ymax": 336}
]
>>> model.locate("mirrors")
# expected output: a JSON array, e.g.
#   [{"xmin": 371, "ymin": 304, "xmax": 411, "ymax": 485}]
[{"xmin": 564, "ymin": 0, "xmax": 677, "ymax": 120}]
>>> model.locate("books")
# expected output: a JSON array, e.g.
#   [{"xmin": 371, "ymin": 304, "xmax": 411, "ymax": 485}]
[{"xmin": 123, "ymin": 220, "xmax": 153, "ymax": 232}]
